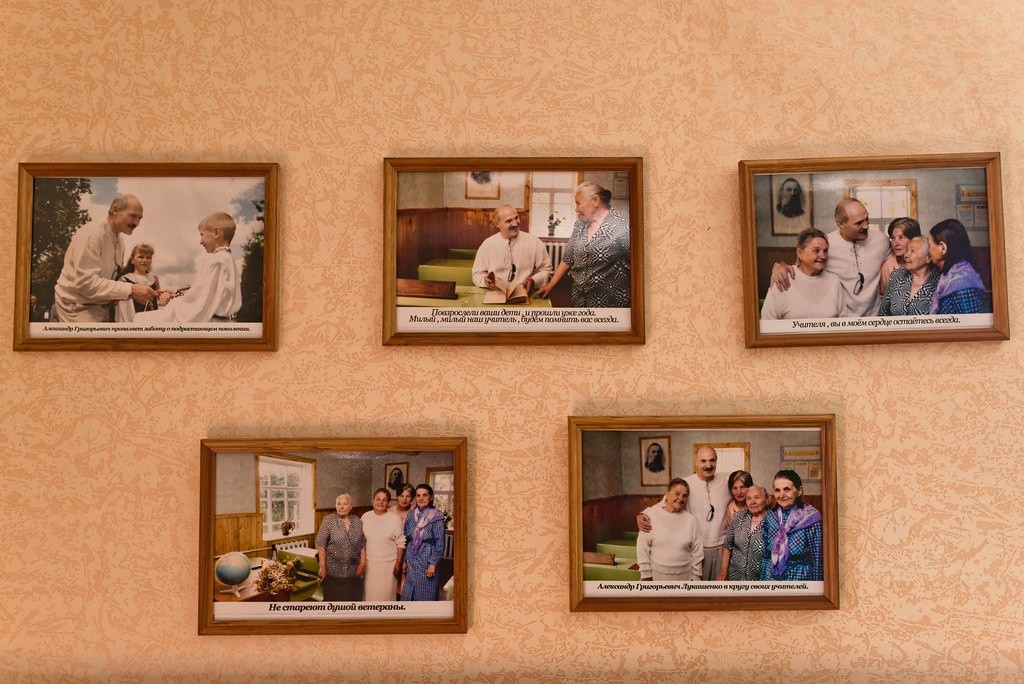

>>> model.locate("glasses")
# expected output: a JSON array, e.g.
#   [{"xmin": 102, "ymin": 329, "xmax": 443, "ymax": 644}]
[
  {"xmin": 706, "ymin": 503, "xmax": 714, "ymax": 522},
  {"xmin": 854, "ymin": 271, "xmax": 864, "ymax": 295},
  {"xmin": 508, "ymin": 262, "xmax": 516, "ymax": 282},
  {"xmin": 112, "ymin": 263, "xmax": 121, "ymax": 281}
]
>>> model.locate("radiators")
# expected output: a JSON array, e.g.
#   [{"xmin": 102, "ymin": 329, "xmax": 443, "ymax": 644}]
[
  {"xmin": 276, "ymin": 539, "xmax": 309, "ymax": 554},
  {"xmin": 543, "ymin": 241, "xmax": 570, "ymax": 275},
  {"xmin": 441, "ymin": 533, "xmax": 455, "ymax": 560}
]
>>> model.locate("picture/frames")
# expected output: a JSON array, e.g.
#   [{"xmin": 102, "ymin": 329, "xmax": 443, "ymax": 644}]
[
  {"xmin": 199, "ymin": 437, "xmax": 468, "ymax": 636},
  {"xmin": 639, "ymin": 436, "xmax": 672, "ymax": 486},
  {"xmin": 384, "ymin": 462, "xmax": 409, "ymax": 500},
  {"xmin": 770, "ymin": 171, "xmax": 813, "ymax": 236},
  {"xmin": 569, "ymin": 414, "xmax": 839, "ymax": 613},
  {"xmin": 738, "ymin": 152, "xmax": 1011, "ymax": 350},
  {"xmin": 10, "ymin": 161, "xmax": 282, "ymax": 354},
  {"xmin": 464, "ymin": 172, "xmax": 500, "ymax": 200},
  {"xmin": 382, "ymin": 156, "xmax": 646, "ymax": 347}
]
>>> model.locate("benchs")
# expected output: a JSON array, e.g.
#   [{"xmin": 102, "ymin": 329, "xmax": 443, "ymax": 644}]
[{"xmin": 273, "ymin": 551, "xmax": 324, "ymax": 601}]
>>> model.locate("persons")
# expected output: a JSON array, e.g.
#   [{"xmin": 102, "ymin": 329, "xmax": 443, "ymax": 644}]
[
  {"xmin": 316, "ymin": 468, "xmax": 445, "ymax": 601},
  {"xmin": 636, "ymin": 443, "xmax": 823, "ymax": 580},
  {"xmin": 472, "ymin": 181, "xmax": 632, "ymax": 307},
  {"xmin": 761, "ymin": 179, "xmax": 988, "ymax": 318},
  {"xmin": 30, "ymin": 193, "xmax": 241, "ymax": 322}
]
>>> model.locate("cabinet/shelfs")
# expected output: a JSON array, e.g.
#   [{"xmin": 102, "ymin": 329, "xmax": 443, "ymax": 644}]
[{"xmin": 213, "ymin": 556, "xmax": 290, "ymax": 603}]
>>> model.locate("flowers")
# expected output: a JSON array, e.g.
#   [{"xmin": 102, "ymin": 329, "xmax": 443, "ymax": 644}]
[
  {"xmin": 279, "ymin": 519, "xmax": 295, "ymax": 535},
  {"xmin": 253, "ymin": 554, "xmax": 304, "ymax": 595},
  {"xmin": 547, "ymin": 210, "xmax": 566, "ymax": 228}
]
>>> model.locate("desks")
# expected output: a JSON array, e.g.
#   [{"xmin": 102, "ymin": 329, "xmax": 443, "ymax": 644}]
[
  {"xmin": 443, "ymin": 576, "xmax": 454, "ymax": 601},
  {"xmin": 583, "ymin": 532, "xmax": 641, "ymax": 582},
  {"xmin": 395, "ymin": 286, "xmax": 553, "ymax": 310},
  {"xmin": 449, "ymin": 249, "xmax": 477, "ymax": 260},
  {"xmin": 418, "ymin": 259, "xmax": 476, "ymax": 286},
  {"xmin": 286, "ymin": 546, "xmax": 318, "ymax": 558}
]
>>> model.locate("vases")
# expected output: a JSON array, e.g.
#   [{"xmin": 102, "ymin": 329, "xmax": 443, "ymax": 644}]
[
  {"xmin": 547, "ymin": 226, "xmax": 554, "ymax": 236},
  {"xmin": 281, "ymin": 530, "xmax": 290, "ymax": 535}
]
